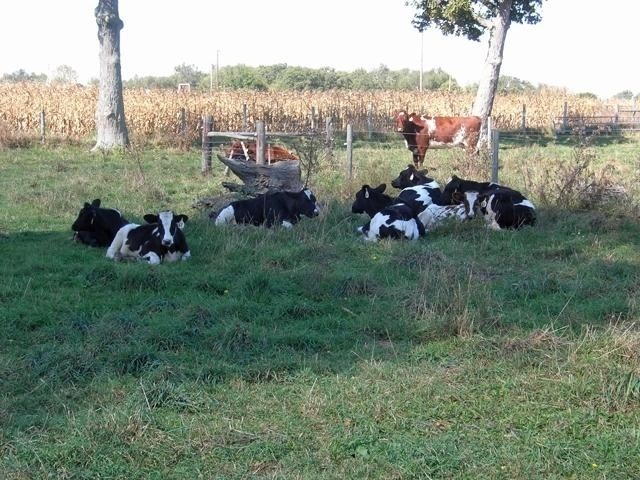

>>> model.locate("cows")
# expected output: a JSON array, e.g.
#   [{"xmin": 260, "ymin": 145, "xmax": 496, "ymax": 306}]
[
  {"xmin": 350, "ymin": 162, "xmax": 541, "ymax": 241},
  {"xmin": 70, "ymin": 197, "xmax": 129, "ymax": 249},
  {"xmin": 392, "ymin": 110, "xmax": 484, "ymax": 169},
  {"xmin": 105, "ymin": 209, "xmax": 194, "ymax": 265},
  {"xmin": 224, "ymin": 142, "xmax": 299, "ymax": 163},
  {"xmin": 213, "ymin": 187, "xmax": 320, "ymax": 229}
]
